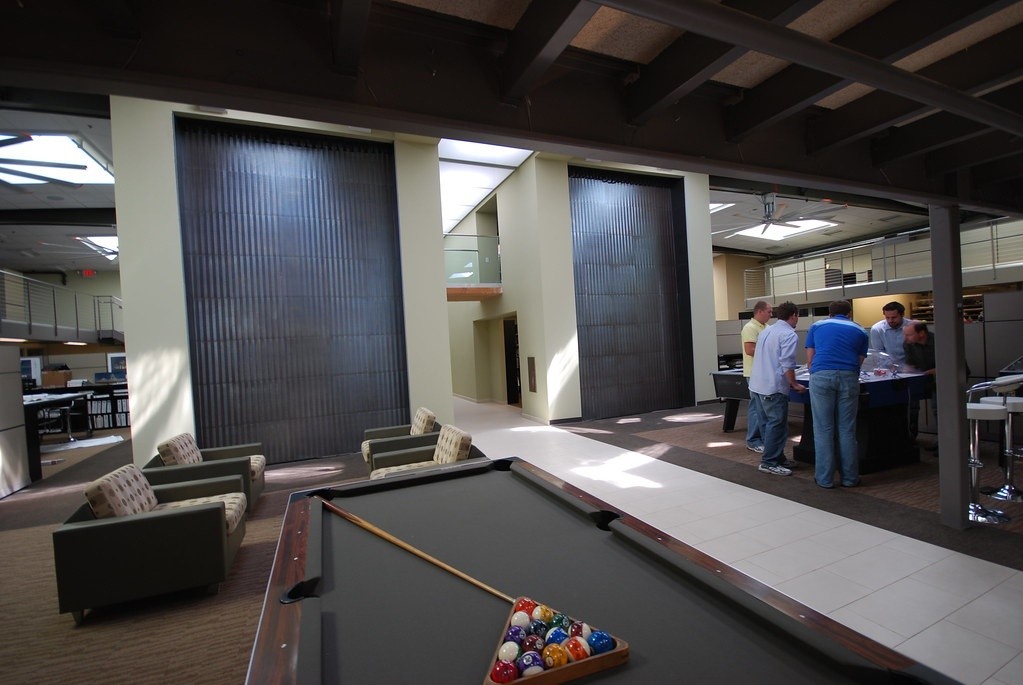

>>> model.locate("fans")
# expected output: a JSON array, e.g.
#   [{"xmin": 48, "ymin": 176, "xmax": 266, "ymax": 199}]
[
  {"xmin": 30, "ymin": 234, "xmax": 121, "ymax": 266},
  {"xmin": 711, "ymin": 193, "xmax": 848, "ymax": 239},
  {"xmin": 0, "ymin": 132, "xmax": 87, "ymax": 192}
]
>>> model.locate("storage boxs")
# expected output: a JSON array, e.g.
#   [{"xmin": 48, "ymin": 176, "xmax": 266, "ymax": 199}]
[{"xmin": 40, "ymin": 370, "xmax": 73, "ymax": 389}]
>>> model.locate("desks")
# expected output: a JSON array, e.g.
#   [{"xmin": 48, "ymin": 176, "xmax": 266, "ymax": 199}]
[
  {"xmin": 709, "ymin": 364, "xmax": 934, "ymax": 476},
  {"xmin": 22, "ymin": 393, "xmax": 88, "ymax": 481},
  {"xmin": 243, "ymin": 455, "xmax": 968, "ymax": 685}
]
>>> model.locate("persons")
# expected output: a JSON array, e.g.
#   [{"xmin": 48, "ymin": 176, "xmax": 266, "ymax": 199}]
[
  {"xmin": 902, "ymin": 323, "xmax": 969, "ymax": 457},
  {"xmin": 747, "ymin": 301, "xmax": 806, "ymax": 476},
  {"xmin": 805, "ymin": 299, "xmax": 869, "ymax": 489},
  {"xmin": 740, "ymin": 301, "xmax": 772, "ymax": 453},
  {"xmin": 871, "ymin": 302, "xmax": 919, "ymax": 440}
]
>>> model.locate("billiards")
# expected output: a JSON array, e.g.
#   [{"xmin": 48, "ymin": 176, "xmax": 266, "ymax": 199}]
[{"xmin": 491, "ymin": 599, "xmax": 613, "ymax": 682}]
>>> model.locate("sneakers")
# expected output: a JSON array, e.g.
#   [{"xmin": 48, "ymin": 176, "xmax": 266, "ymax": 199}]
[
  {"xmin": 758, "ymin": 464, "xmax": 792, "ymax": 475},
  {"xmin": 746, "ymin": 444, "xmax": 764, "ymax": 453}
]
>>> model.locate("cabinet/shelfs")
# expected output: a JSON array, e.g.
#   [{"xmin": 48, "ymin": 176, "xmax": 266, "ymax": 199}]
[{"xmin": 26, "ymin": 382, "xmax": 132, "ymax": 434}]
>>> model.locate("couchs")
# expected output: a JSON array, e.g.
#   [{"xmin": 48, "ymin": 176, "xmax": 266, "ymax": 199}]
[
  {"xmin": 50, "ymin": 464, "xmax": 248, "ymax": 627},
  {"xmin": 360, "ymin": 406, "xmax": 443, "ymax": 473},
  {"xmin": 141, "ymin": 432, "xmax": 266, "ymax": 514},
  {"xmin": 370, "ymin": 424, "xmax": 486, "ymax": 481}
]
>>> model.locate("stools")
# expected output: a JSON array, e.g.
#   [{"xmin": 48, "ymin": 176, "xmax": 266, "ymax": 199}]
[{"xmin": 965, "ymin": 373, "xmax": 1023, "ymax": 524}]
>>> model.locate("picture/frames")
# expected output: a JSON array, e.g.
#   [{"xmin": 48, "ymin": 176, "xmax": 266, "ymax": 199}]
[{"xmin": 106, "ymin": 351, "xmax": 127, "ymax": 374}]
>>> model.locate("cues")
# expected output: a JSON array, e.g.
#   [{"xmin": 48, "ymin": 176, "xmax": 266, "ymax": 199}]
[{"xmin": 312, "ymin": 492, "xmax": 516, "ymax": 604}]
